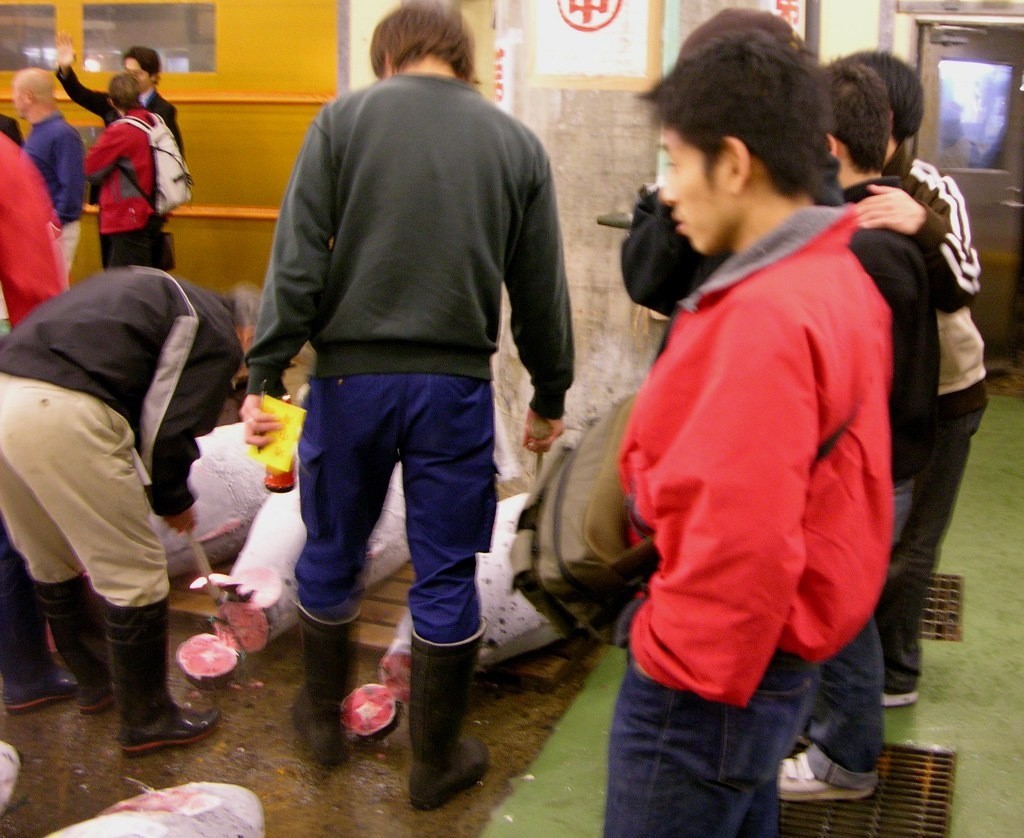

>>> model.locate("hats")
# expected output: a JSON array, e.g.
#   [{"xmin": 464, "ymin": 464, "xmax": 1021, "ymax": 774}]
[{"xmin": 675, "ymin": 5, "xmax": 817, "ymax": 67}]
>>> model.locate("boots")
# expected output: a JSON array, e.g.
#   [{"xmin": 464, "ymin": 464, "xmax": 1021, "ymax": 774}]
[
  {"xmin": 103, "ymin": 592, "xmax": 220, "ymax": 750},
  {"xmin": 32, "ymin": 572, "xmax": 119, "ymax": 712},
  {"xmin": 0, "ymin": 557, "xmax": 80, "ymax": 715},
  {"xmin": 290, "ymin": 599, "xmax": 361, "ymax": 766},
  {"xmin": 408, "ymin": 617, "xmax": 491, "ymax": 809}
]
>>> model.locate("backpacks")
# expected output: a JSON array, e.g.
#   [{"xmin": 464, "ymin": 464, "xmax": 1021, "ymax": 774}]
[{"xmin": 108, "ymin": 113, "xmax": 195, "ymax": 214}]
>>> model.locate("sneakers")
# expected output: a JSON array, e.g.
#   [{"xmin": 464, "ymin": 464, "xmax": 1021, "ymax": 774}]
[{"xmin": 771, "ymin": 752, "xmax": 876, "ymax": 801}]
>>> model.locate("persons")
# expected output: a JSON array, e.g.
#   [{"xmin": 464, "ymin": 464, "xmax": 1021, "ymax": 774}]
[
  {"xmin": 775, "ymin": 49, "xmax": 991, "ymax": 803},
  {"xmin": 602, "ymin": 8, "xmax": 901, "ymax": 838},
  {"xmin": 242, "ymin": 0, "xmax": 576, "ymax": 812},
  {"xmin": 1, "ymin": 266, "xmax": 263, "ymax": 755},
  {"xmin": 12, "ymin": 66, "xmax": 87, "ymax": 292},
  {"xmin": 84, "ymin": 74, "xmax": 165, "ymax": 273},
  {"xmin": 0, "ymin": 114, "xmax": 71, "ymax": 332},
  {"xmin": 56, "ymin": 30, "xmax": 184, "ymax": 230}
]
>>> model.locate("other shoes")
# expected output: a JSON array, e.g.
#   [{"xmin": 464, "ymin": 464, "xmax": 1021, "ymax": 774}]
[{"xmin": 880, "ymin": 672, "xmax": 920, "ymax": 707}]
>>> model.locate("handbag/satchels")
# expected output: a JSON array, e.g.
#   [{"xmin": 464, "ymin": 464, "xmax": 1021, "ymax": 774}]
[
  {"xmin": 107, "ymin": 231, "xmax": 177, "ymax": 271},
  {"xmin": 510, "ymin": 393, "xmax": 662, "ymax": 650}
]
